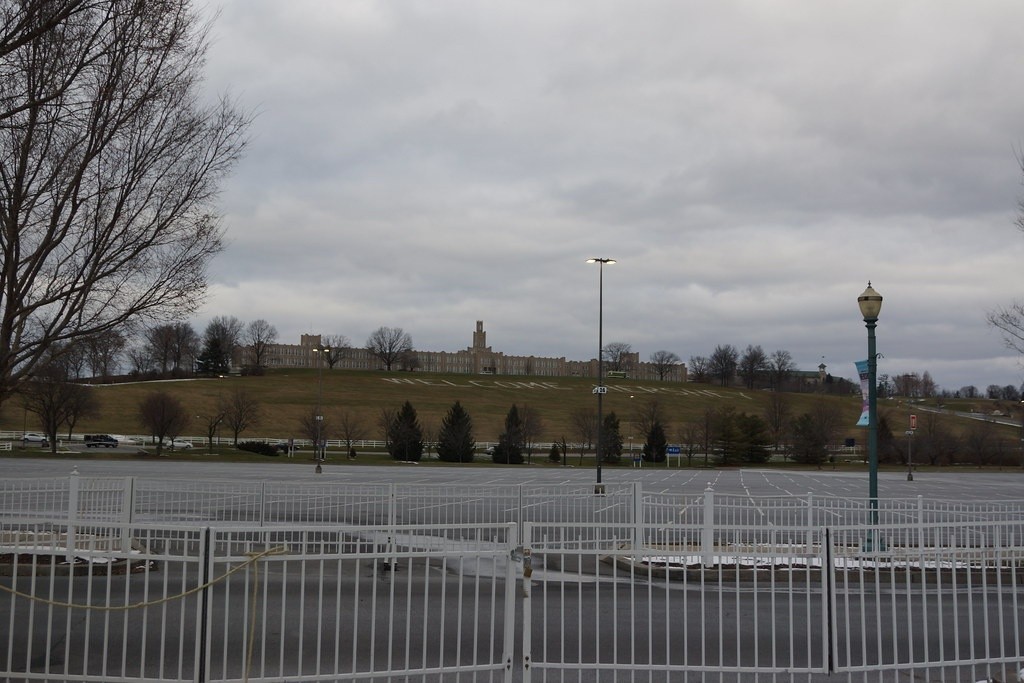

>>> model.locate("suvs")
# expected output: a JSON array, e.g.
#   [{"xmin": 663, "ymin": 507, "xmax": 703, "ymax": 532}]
[{"xmin": 84, "ymin": 435, "xmax": 118, "ymax": 448}]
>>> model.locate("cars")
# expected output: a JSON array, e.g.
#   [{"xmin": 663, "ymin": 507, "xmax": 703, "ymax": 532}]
[
  {"xmin": 167, "ymin": 440, "xmax": 194, "ymax": 450},
  {"xmin": 21, "ymin": 432, "xmax": 50, "ymax": 442}
]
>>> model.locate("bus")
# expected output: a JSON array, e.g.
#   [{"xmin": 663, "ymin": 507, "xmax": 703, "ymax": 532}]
[{"xmin": 607, "ymin": 371, "xmax": 627, "ymax": 379}]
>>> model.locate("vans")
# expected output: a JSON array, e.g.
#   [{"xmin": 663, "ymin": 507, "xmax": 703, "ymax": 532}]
[{"xmin": 480, "ymin": 371, "xmax": 493, "ymax": 375}]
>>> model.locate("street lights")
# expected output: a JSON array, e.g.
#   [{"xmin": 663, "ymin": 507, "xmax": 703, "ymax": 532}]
[
  {"xmin": 315, "ymin": 415, "xmax": 324, "ymax": 473},
  {"xmin": 313, "ymin": 347, "xmax": 328, "ymax": 413},
  {"xmin": 857, "ymin": 281, "xmax": 888, "ymax": 551},
  {"xmin": 586, "ymin": 259, "xmax": 618, "ymax": 496}
]
